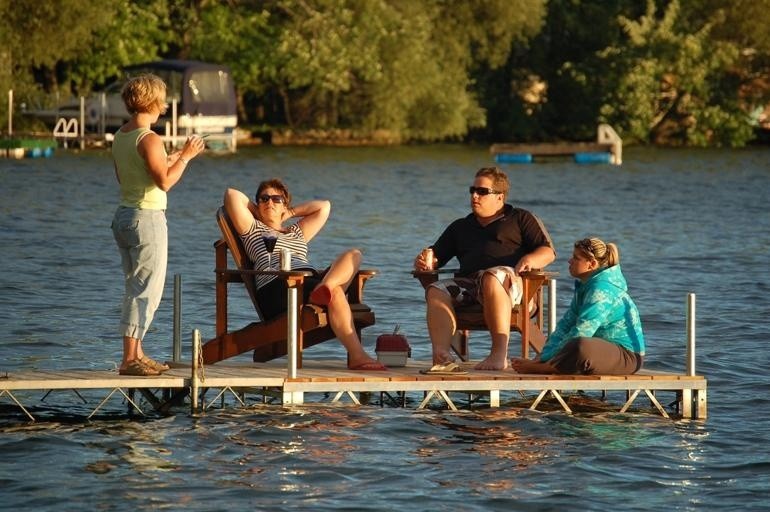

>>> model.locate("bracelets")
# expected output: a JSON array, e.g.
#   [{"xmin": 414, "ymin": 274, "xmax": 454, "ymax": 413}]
[
  {"xmin": 288, "ymin": 208, "xmax": 295, "ymax": 217},
  {"xmin": 178, "ymin": 157, "xmax": 188, "ymax": 167}
]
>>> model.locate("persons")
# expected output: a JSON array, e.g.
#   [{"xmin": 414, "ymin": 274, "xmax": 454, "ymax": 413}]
[
  {"xmin": 415, "ymin": 165, "xmax": 556, "ymax": 370},
  {"xmin": 107, "ymin": 73, "xmax": 204, "ymax": 376},
  {"xmin": 518, "ymin": 237, "xmax": 645, "ymax": 375},
  {"xmin": 223, "ymin": 179, "xmax": 388, "ymax": 371}
]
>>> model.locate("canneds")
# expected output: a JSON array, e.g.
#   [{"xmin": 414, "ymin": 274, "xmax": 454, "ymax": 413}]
[{"xmin": 422, "ymin": 248, "xmax": 434, "ymax": 270}]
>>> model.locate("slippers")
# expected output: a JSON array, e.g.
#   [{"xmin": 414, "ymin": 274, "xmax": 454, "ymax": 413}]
[
  {"xmin": 119, "ymin": 355, "xmax": 169, "ymax": 375},
  {"xmin": 420, "ymin": 361, "xmax": 468, "ymax": 374},
  {"xmin": 310, "ymin": 285, "xmax": 331, "ymax": 306},
  {"xmin": 349, "ymin": 362, "xmax": 385, "ymax": 370}
]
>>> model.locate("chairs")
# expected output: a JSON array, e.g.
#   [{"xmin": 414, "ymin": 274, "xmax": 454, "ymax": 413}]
[
  {"xmin": 410, "ymin": 257, "xmax": 558, "ymax": 364},
  {"xmin": 202, "ymin": 198, "xmax": 379, "ymax": 378}
]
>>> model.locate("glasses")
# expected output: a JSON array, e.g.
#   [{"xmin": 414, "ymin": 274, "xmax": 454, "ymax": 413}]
[
  {"xmin": 257, "ymin": 194, "xmax": 283, "ymax": 203},
  {"xmin": 470, "ymin": 186, "xmax": 493, "ymax": 194},
  {"xmin": 583, "ymin": 238, "xmax": 594, "ymax": 254}
]
